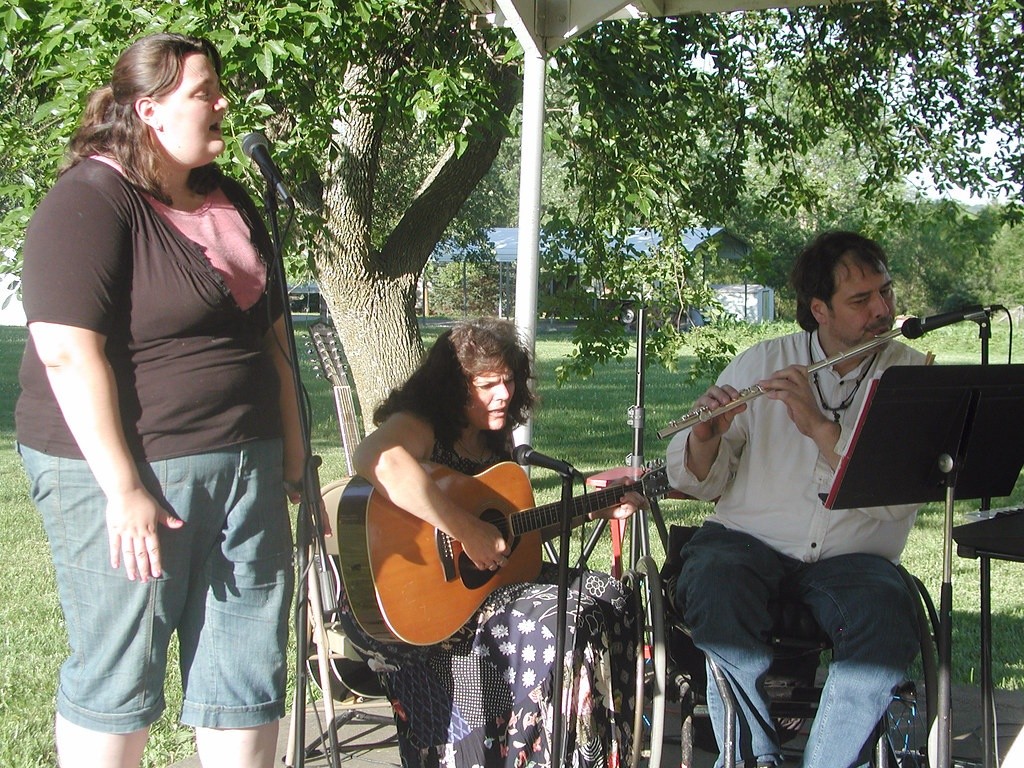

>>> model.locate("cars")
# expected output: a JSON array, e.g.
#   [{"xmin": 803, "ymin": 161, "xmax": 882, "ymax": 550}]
[{"xmin": 290, "ymin": 292, "xmax": 320, "ymax": 314}]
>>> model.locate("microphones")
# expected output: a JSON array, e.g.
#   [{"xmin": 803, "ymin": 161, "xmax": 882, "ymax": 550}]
[
  {"xmin": 241, "ymin": 133, "xmax": 294, "ymax": 208},
  {"xmin": 902, "ymin": 305, "xmax": 1004, "ymax": 340},
  {"xmin": 512, "ymin": 444, "xmax": 583, "ymax": 479}
]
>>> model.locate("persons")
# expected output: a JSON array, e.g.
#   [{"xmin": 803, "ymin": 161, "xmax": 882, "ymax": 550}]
[
  {"xmin": 337, "ymin": 315, "xmax": 650, "ymax": 768},
  {"xmin": 665, "ymin": 230, "xmax": 942, "ymax": 768},
  {"xmin": 16, "ymin": 33, "xmax": 334, "ymax": 768}
]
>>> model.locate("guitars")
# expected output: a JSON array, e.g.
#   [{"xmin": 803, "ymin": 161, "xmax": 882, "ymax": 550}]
[
  {"xmin": 335, "ymin": 457, "xmax": 674, "ymax": 647},
  {"xmin": 300, "ymin": 320, "xmax": 387, "ymax": 705}
]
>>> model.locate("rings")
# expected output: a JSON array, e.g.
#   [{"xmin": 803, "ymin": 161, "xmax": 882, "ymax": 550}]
[
  {"xmin": 488, "ymin": 564, "xmax": 496, "ymax": 569},
  {"xmin": 497, "ymin": 557, "xmax": 506, "ymax": 565}
]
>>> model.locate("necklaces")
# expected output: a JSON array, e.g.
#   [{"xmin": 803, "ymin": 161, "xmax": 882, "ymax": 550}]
[{"xmin": 809, "ymin": 330, "xmax": 877, "ymax": 422}]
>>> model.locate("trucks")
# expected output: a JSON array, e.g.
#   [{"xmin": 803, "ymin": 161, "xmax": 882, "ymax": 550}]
[{"xmin": 539, "ymin": 275, "xmax": 666, "ymax": 326}]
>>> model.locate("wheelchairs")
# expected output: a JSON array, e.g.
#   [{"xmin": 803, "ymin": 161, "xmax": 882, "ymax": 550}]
[{"xmin": 617, "ymin": 525, "xmax": 939, "ymax": 768}]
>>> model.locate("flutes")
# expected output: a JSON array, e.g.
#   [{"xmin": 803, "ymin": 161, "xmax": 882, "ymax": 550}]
[{"xmin": 655, "ymin": 326, "xmax": 904, "ymax": 443}]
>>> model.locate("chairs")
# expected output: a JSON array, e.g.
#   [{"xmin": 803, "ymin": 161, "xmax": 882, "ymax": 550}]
[{"xmin": 284, "ymin": 479, "xmax": 397, "ymax": 768}]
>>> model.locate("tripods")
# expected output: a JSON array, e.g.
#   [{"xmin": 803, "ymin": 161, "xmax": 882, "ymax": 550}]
[{"xmin": 576, "ymin": 307, "xmax": 669, "ymax": 579}]
